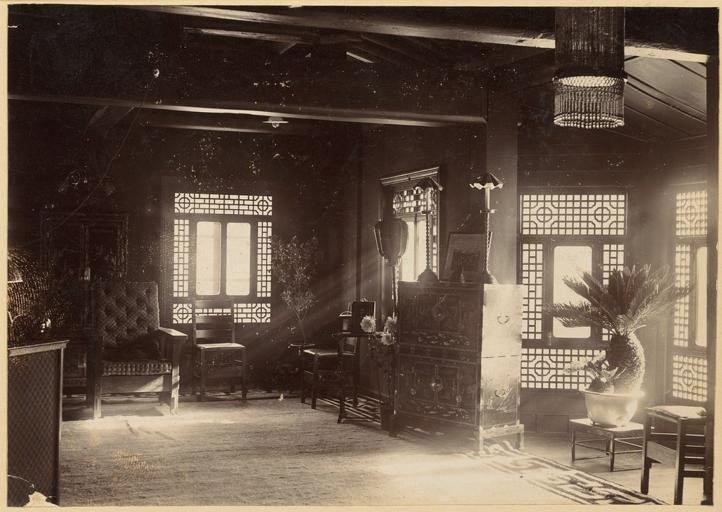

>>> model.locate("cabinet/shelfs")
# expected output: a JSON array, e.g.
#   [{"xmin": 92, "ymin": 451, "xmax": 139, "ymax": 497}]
[{"xmin": 388, "ymin": 281, "xmax": 525, "ymax": 456}]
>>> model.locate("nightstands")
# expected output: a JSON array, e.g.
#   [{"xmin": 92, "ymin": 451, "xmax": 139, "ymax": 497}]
[{"xmin": 570, "ymin": 418, "xmax": 652, "ymax": 473}]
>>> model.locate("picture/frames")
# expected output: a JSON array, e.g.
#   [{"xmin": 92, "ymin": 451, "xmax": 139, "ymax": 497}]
[{"xmin": 439, "ymin": 231, "xmax": 493, "ymax": 286}]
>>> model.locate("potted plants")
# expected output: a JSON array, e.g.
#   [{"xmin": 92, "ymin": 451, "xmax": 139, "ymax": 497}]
[
  {"xmin": 544, "ymin": 264, "xmax": 696, "ymax": 429},
  {"xmin": 268, "ymin": 230, "xmax": 319, "ymax": 388}
]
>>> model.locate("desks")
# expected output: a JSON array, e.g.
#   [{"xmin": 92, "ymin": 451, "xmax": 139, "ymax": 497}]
[
  {"xmin": 332, "ymin": 332, "xmax": 398, "ymax": 424},
  {"xmin": 641, "ymin": 406, "xmax": 709, "ymax": 505}
]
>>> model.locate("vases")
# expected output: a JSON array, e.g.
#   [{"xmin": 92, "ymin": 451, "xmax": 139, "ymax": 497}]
[{"xmin": 380, "ymin": 403, "xmax": 394, "ymax": 431}]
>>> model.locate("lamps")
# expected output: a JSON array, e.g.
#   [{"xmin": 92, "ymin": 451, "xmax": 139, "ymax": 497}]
[
  {"xmin": 374, "ymin": 215, "xmax": 408, "ymax": 317},
  {"xmin": 412, "ymin": 176, "xmax": 444, "ymax": 284},
  {"xmin": 552, "ymin": 7, "xmax": 628, "ymax": 131},
  {"xmin": 470, "ymin": 172, "xmax": 505, "ymax": 286}
]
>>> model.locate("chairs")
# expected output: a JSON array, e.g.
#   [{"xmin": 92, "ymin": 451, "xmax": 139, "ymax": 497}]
[
  {"xmin": 191, "ymin": 297, "xmax": 248, "ymax": 402},
  {"xmin": 86, "ymin": 280, "xmax": 189, "ymax": 419},
  {"xmin": 288, "ymin": 300, "xmax": 377, "ymax": 409}
]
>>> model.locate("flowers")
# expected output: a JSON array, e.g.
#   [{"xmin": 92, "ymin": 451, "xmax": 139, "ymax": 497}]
[{"xmin": 360, "ymin": 311, "xmax": 398, "ymax": 409}]
